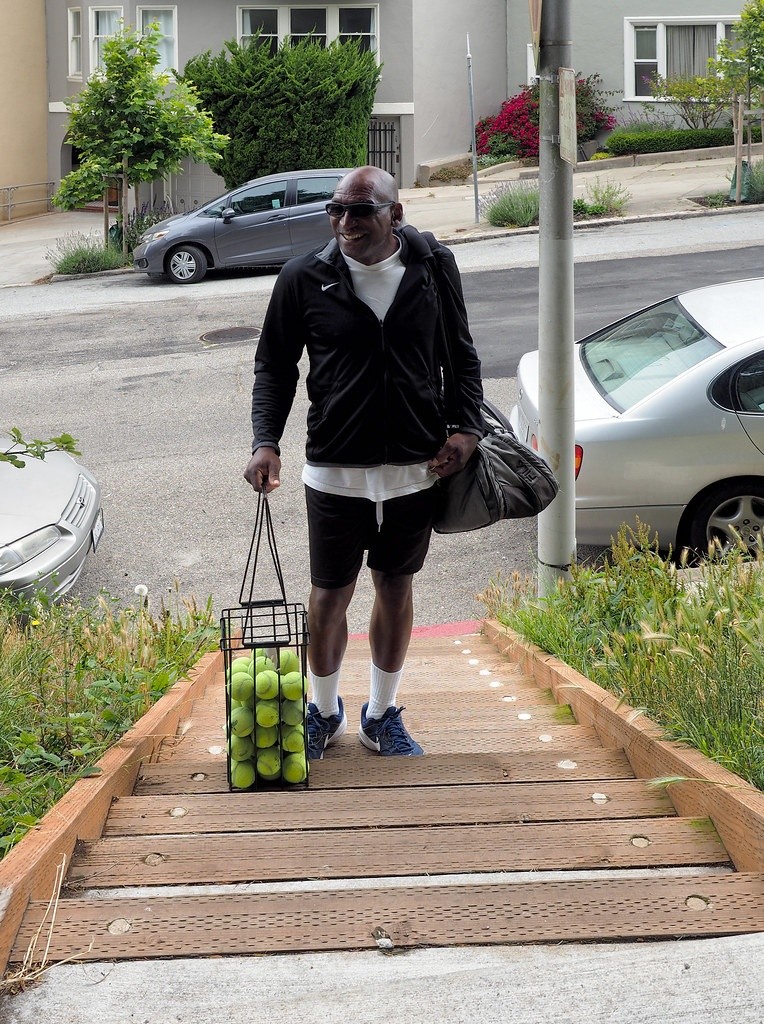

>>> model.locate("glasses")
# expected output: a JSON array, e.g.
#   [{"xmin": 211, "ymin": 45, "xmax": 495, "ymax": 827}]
[{"xmin": 325, "ymin": 200, "xmax": 395, "ymax": 219}]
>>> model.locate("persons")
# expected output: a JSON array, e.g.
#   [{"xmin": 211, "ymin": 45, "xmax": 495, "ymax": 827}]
[{"xmin": 244, "ymin": 165, "xmax": 487, "ymax": 762}]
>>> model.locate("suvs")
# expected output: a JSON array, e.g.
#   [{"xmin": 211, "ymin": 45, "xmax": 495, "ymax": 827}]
[{"xmin": 130, "ymin": 167, "xmax": 355, "ymax": 284}]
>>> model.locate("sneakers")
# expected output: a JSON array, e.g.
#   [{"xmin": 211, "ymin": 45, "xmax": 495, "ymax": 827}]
[
  {"xmin": 305, "ymin": 693, "xmax": 347, "ymax": 759},
  {"xmin": 360, "ymin": 703, "xmax": 425, "ymax": 758}
]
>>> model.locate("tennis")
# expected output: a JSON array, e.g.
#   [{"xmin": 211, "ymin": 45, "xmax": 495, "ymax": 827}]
[{"xmin": 228, "ymin": 648, "xmax": 307, "ymax": 787}]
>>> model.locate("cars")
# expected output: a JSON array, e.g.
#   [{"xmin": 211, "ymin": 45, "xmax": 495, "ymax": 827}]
[
  {"xmin": 0, "ymin": 437, "xmax": 106, "ymax": 635},
  {"xmin": 508, "ymin": 275, "xmax": 764, "ymax": 569}
]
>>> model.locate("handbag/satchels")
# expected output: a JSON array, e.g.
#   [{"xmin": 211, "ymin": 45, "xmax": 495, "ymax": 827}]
[{"xmin": 431, "ymin": 387, "xmax": 560, "ymax": 535}]
